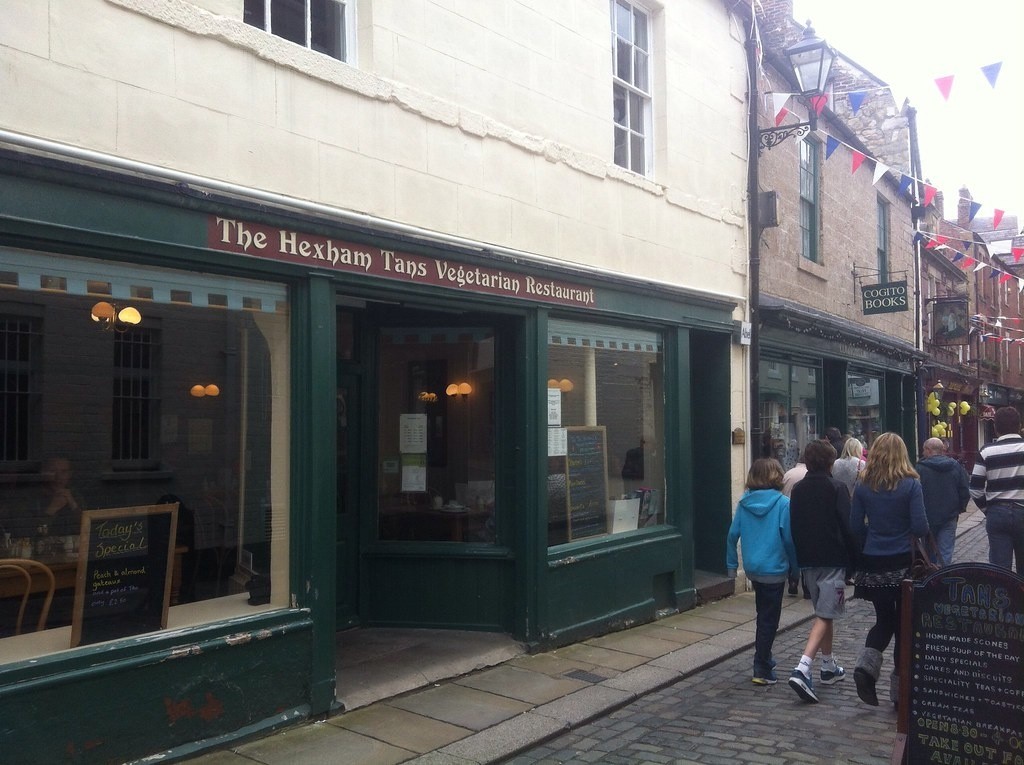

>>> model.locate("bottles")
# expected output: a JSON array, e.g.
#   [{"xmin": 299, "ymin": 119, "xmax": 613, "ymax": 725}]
[{"xmin": 34, "ymin": 523, "xmax": 50, "ymax": 561}]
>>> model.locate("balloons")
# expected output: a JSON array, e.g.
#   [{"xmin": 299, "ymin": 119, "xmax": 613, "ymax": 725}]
[{"xmin": 926, "ymin": 391, "xmax": 970, "ymax": 437}]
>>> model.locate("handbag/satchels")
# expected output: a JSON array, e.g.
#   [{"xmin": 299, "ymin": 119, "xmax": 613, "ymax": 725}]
[
  {"xmin": 909, "ymin": 531, "xmax": 944, "ymax": 583},
  {"xmin": 853, "ymin": 568, "xmax": 909, "ymax": 598}
]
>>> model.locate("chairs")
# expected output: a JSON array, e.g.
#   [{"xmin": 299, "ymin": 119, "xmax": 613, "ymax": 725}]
[{"xmin": 0, "ymin": 559, "xmax": 57, "ymax": 640}]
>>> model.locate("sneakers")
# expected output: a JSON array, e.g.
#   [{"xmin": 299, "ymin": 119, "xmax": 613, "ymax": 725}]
[
  {"xmin": 820, "ymin": 664, "xmax": 845, "ymax": 685},
  {"xmin": 789, "ymin": 669, "xmax": 819, "ymax": 703},
  {"xmin": 751, "ymin": 670, "xmax": 777, "ymax": 684},
  {"xmin": 771, "ymin": 660, "xmax": 776, "ymax": 669}
]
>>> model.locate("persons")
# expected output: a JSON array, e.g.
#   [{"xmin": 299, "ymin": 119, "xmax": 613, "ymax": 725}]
[
  {"xmin": 35, "ymin": 453, "xmax": 85, "ymax": 538},
  {"xmin": 971, "ymin": 411, "xmax": 1024, "ymax": 578},
  {"xmin": 915, "ymin": 437, "xmax": 970, "ymax": 568},
  {"xmin": 849, "ymin": 433, "xmax": 929, "ymax": 710},
  {"xmin": 824, "ymin": 428, "xmax": 868, "ymax": 585},
  {"xmin": 788, "ymin": 439, "xmax": 851, "ymax": 703},
  {"xmin": 727, "ymin": 458, "xmax": 799, "ymax": 685},
  {"xmin": 783, "ymin": 455, "xmax": 812, "ymax": 599}
]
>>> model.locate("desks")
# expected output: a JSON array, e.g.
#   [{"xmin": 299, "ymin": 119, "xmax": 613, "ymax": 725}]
[
  {"xmin": 385, "ymin": 503, "xmax": 490, "ymax": 545},
  {"xmin": 0, "ymin": 541, "xmax": 189, "ymax": 610}
]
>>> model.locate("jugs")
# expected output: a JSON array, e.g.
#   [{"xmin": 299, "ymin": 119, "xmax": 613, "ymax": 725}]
[{"xmin": 431, "ymin": 494, "xmax": 443, "ymax": 511}]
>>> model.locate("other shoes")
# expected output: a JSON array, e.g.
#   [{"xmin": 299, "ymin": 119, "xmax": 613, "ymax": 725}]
[
  {"xmin": 854, "ymin": 668, "xmax": 879, "ymax": 706},
  {"xmin": 802, "ymin": 587, "xmax": 811, "ymax": 599},
  {"xmin": 788, "ymin": 580, "xmax": 799, "ymax": 595}
]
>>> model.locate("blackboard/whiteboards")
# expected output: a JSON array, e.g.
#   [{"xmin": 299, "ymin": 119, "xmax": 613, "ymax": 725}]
[{"xmin": 71, "ymin": 503, "xmax": 179, "ymax": 648}]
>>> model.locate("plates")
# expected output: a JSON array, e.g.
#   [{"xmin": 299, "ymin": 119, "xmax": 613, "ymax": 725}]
[{"xmin": 441, "ymin": 508, "xmax": 470, "ymax": 513}]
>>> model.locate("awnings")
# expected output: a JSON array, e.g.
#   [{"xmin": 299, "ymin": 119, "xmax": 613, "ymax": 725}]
[{"xmin": 978, "ymin": 405, "xmax": 997, "ymax": 422}]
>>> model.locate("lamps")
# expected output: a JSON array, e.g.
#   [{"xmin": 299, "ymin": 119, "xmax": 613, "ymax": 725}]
[
  {"xmin": 446, "ymin": 383, "xmax": 472, "ymax": 409},
  {"xmin": 758, "ymin": 18, "xmax": 835, "ymax": 156},
  {"xmin": 89, "ymin": 297, "xmax": 141, "ymax": 334},
  {"xmin": 548, "ymin": 378, "xmax": 574, "ymax": 397},
  {"xmin": 190, "ymin": 384, "xmax": 220, "ymax": 398}
]
